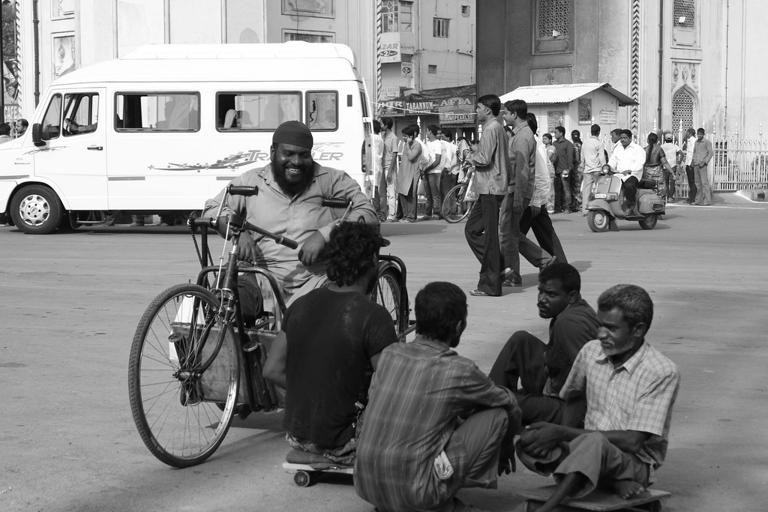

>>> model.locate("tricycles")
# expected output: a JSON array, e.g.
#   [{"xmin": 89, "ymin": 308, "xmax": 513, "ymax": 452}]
[{"xmin": 121, "ymin": 180, "xmax": 420, "ymax": 476}]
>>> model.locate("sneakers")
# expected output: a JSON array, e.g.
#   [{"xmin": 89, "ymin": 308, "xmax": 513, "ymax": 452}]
[{"xmin": 378, "ymin": 214, "xmax": 442, "ymax": 223}]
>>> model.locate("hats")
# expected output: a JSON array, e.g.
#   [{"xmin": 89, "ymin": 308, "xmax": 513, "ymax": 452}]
[{"xmin": 272, "ymin": 121, "xmax": 313, "ymax": 150}]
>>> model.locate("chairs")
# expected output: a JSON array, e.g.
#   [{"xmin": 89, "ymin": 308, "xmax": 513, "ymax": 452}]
[{"xmin": 223, "ymin": 109, "xmax": 238, "ymax": 129}]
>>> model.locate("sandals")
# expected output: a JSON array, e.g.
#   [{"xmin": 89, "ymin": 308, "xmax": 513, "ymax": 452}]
[
  {"xmin": 470, "ymin": 289, "xmax": 488, "ymax": 296},
  {"xmin": 539, "ymin": 256, "xmax": 557, "ymax": 271},
  {"xmin": 502, "ymin": 279, "xmax": 522, "ymax": 287}
]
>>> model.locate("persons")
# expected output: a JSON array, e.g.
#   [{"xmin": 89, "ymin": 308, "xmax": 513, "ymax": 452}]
[
  {"xmin": 262, "ymin": 221, "xmax": 397, "ymax": 468},
  {"xmin": 154, "ymin": 100, "xmax": 174, "ymax": 130},
  {"xmin": 497, "ymin": 100, "xmax": 555, "ymax": 287},
  {"xmin": 202, "ymin": 121, "xmax": 378, "ymax": 325},
  {"xmin": 354, "ymin": 281, "xmax": 523, "ymax": 511},
  {"xmin": 460, "ymin": 262, "xmax": 602, "ymax": 433},
  {"xmin": 370, "ymin": 117, "xmax": 483, "ymax": 222},
  {"xmin": 542, "ymin": 123, "xmax": 714, "ymax": 214},
  {"xmin": 501, "ymin": 112, "xmax": 569, "ymax": 271},
  {"xmin": 75, "ymin": 209, "xmax": 199, "ymax": 227},
  {"xmin": 0, "ymin": 119, "xmax": 28, "ymax": 143},
  {"xmin": 73, "ymin": 112, "xmax": 119, "ymax": 132},
  {"xmin": 501, "ymin": 284, "xmax": 679, "ymax": 511},
  {"xmin": 462, "ymin": 93, "xmax": 508, "ymax": 295}
]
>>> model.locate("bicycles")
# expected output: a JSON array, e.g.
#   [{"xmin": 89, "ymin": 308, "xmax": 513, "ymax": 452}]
[{"xmin": 439, "ymin": 162, "xmax": 476, "ymax": 224}]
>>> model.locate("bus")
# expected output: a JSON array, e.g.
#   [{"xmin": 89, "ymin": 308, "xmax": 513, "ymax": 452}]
[{"xmin": 0, "ymin": 36, "xmax": 383, "ymax": 235}]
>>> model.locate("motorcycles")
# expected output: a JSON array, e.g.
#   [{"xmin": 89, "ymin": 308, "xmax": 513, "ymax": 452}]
[{"xmin": 583, "ymin": 164, "xmax": 667, "ymax": 234}]
